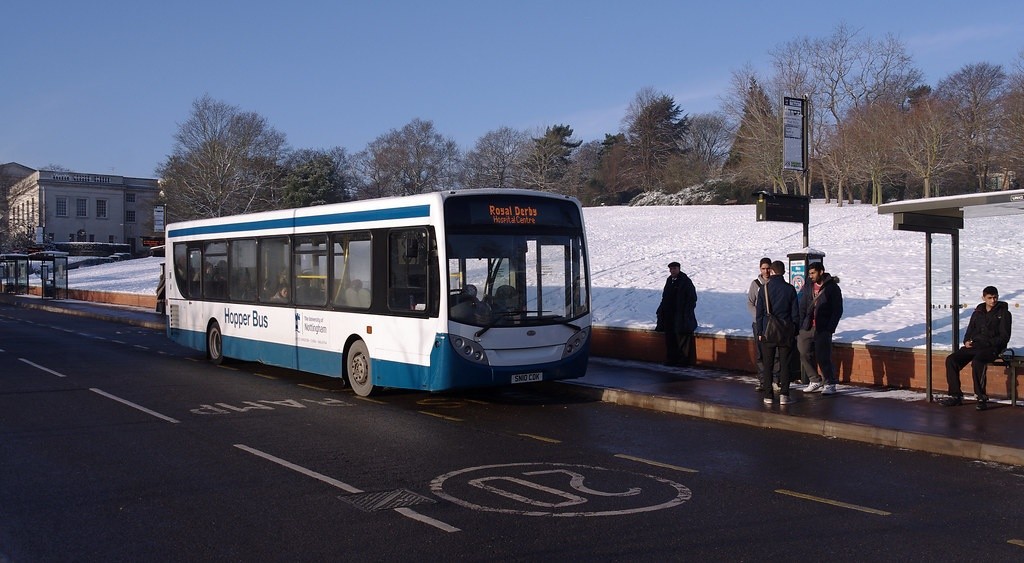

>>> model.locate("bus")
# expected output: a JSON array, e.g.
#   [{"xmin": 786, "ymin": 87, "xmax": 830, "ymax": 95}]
[{"xmin": 153, "ymin": 185, "xmax": 593, "ymax": 403}]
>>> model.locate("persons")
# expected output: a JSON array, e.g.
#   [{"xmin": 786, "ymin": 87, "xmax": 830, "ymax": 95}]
[
  {"xmin": 756, "ymin": 261, "xmax": 799, "ymax": 405},
  {"xmin": 178, "ymin": 256, "xmax": 194, "ymax": 280},
  {"xmin": 944, "ymin": 287, "xmax": 1011, "ymax": 409},
  {"xmin": 193, "ymin": 261, "xmax": 212, "ymax": 281},
  {"xmin": 655, "ymin": 262, "xmax": 698, "ymax": 367},
  {"xmin": 450, "ymin": 285, "xmax": 479, "ymax": 306},
  {"xmin": 748, "ymin": 258, "xmax": 782, "ymax": 391},
  {"xmin": 270, "ymin": 284, "xmax": 290, "ymax": 304},
  {"xmin": 796, "ymin": 262, "xmax": 843, "ymax": 394}
]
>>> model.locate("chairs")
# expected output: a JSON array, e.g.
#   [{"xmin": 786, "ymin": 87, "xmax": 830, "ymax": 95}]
[
  {"xmin": 345, "ymin": 288, "xmax": 360, "ymax": 307},
  {"xmin": 493, "ymin": 285, "xmax": 519, "ymax": 315},
  {"xmin": 456, "ymin": 285, "xmax": 479, "ymax": 306},
  {"xmin": 358, "ymin": 288, "xmax": 371, "ymax": 308}
]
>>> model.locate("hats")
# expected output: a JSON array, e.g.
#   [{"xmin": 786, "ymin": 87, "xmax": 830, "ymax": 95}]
[{"xmin": 668, "ymin": 262, "xmax": 680, "ymax": 267}]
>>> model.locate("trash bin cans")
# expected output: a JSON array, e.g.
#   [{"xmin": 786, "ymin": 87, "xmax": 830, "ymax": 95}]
[{"xmin": 43, "ymin": 279, "xmax": 53, "ymax": 297}]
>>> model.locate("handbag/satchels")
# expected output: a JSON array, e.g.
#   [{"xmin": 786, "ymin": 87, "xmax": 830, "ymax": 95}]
[
  {"xmin": 764, "ymin": 314, "xmax": 796, "ymax": 347},
  {"xmin": 803, "ymin": 310, "xmax": 814, "ymax": 330}
]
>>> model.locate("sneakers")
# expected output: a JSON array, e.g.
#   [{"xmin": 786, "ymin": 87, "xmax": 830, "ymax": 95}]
[
  {"xmin": 779, "ymin": 395, "xmax": 796, "ymax": 404},
  {"xmin": 976, "ymin": 396, "xmax": 988, "ymax": 408},
  {"xmin": 756, "ymin": 383, "xmax": 768, "ymax": 392},
  {"xmin": 938, "ymin": 396, "xmax": 962, "ymax": 406},
  {"xmin": 802, "ymin": 381, "xmax": 822, "ymax": 392},
  {"xmin": 763, "ymin": 398, "xmax": 773, "ymax": 404},
  {"xmin": 821, "ymin": 384, "xmax": 836, "ymax": 394}
]
